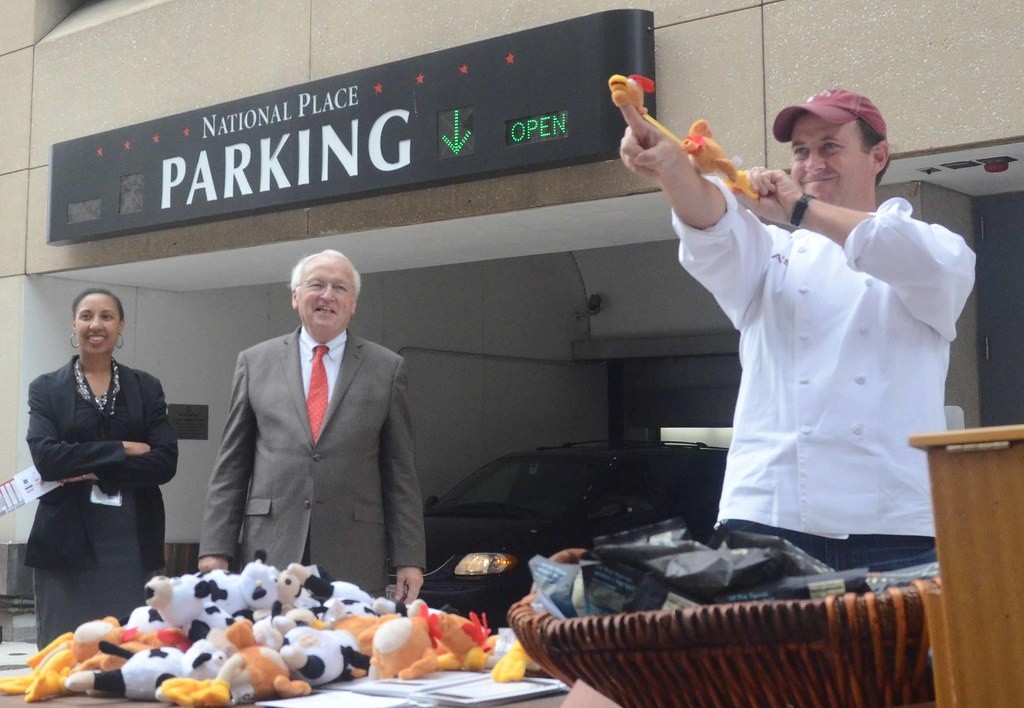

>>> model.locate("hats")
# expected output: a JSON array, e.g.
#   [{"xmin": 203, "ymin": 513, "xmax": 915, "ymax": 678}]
[{"xmin": 773, "ymin": 89, "xmax": 887, "ymax": 142}]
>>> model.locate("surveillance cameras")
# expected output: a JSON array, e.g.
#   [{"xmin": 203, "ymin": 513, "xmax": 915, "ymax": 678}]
[{"xmin": 586, "ymin": 295, "xmax": 602, "ymax": 316}]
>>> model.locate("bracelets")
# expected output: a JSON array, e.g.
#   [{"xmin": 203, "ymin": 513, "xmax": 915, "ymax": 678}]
[
  {"xmin": 82, "ymin": 474, "xmax": 86, "ymax": 479},
  {"xmin": 790, "ymin": 193, "xmax": 815, "ymax": 226}
]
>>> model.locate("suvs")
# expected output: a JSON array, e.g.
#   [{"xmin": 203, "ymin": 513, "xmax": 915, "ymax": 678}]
[{"xmin": 386, "ymin": 438, "xmax": 730, "ymax": 637}]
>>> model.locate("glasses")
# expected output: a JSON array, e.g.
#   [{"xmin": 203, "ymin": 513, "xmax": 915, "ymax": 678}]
[{"xmin": 298, "ymin": 280, "xmax": 355, "ymax": 294}]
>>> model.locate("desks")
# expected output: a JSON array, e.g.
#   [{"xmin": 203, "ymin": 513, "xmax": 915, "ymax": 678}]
[{"xmin": 0, "ymin": 668, "xmax": 933, "ymax": 708}]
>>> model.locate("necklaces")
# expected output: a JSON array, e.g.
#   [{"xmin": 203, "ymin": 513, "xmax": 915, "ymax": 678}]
[{"xmin": 73, "ymin": 358, "xmax": 120, "ymax": 415}]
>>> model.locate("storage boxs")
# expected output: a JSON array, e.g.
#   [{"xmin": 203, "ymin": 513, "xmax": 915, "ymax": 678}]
[{"xmin": 506, "ymin": 577, "xmax": 937, "ymax": 708}]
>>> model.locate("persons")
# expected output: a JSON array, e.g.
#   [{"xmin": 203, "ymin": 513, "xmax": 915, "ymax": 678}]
[
  {"xmin": 198, "ymin": 249, "xmax": 428, "ymax": 604},
  {"xmin": 22, "ymin": 287, "xmax": 179, "ymax": 651},
  {"xmin": 620, "ymin": 88, "xmax": 977, "ymax": 574}
]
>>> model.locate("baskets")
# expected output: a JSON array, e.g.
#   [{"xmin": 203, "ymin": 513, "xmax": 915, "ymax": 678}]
[{"xmin": 508, "ymin": 550, "xmax": 939, "ymax": 708}]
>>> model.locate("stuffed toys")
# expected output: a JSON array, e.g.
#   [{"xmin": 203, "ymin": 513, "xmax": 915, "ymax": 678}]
[
  {"xmin": 0, "ymin": 549, "xmax": 532, "ymax": 708},
  {"xmin": 608, "ymin": 74, "xmax": 760, "ymax": 198}
]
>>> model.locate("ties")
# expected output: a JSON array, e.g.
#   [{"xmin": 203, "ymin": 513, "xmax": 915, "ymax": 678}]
[{"xmin": 306, "ymin": 346, "xmax": 329, "ymax": 443}]
